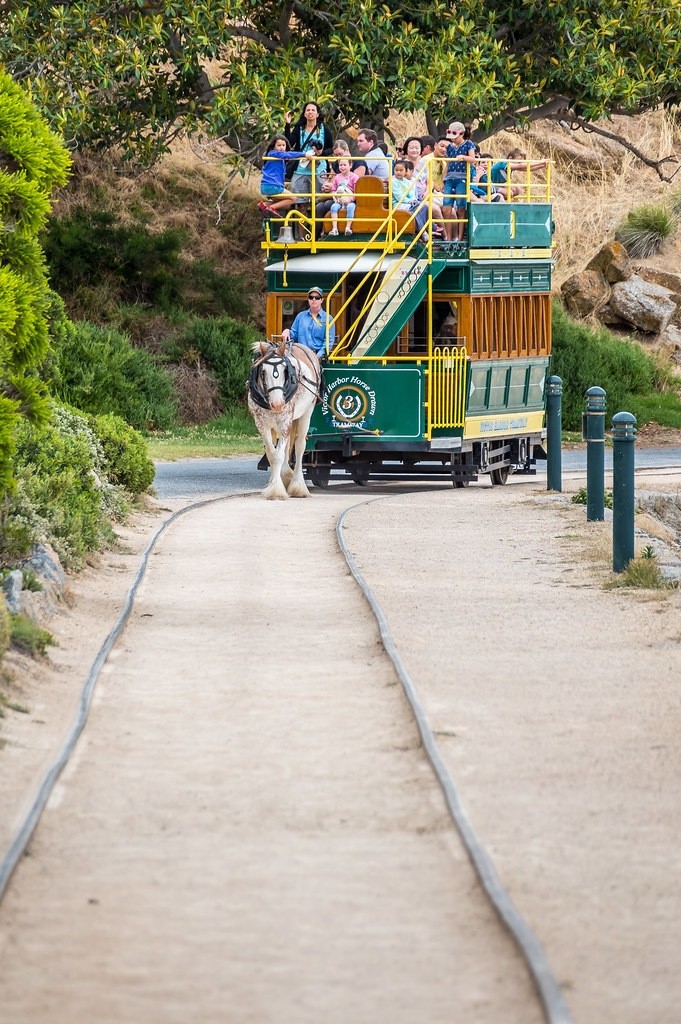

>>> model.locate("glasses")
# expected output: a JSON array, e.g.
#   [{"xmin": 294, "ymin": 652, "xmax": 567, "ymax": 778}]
[
  {"xmin": 396, "ymin": 147, "xmax": 403, "ymax": 152},
  {"xmin": 309, "ymin": 296, "xmax": 322, "ymax": 300},
  {"xmin": 446, "ymin": 129, "xmax": 462, "ymax": 135}
]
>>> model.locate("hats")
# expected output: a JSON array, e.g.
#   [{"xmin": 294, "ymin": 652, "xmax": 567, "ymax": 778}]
[
  {"xmin": 443, "ymin": 316, "xmax": 458, "ymax": 324},
  {"xmin": 308, "ymin": 286, "xmax": 323, "ymax": 295},
  {"xmin": 446, "ymin": 122, "xmax": 466, "ymax": 138}
]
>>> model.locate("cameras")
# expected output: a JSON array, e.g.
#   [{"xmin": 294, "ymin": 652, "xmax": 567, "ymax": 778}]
[{"xmin": 308, "ymin": 138, "xmax": 322, "ymax": 150}]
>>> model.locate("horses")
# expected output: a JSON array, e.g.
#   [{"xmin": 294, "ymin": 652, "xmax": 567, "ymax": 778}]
[{"xmin": 244, "ymin": 340, "xmax": 324, "ymax": 500}]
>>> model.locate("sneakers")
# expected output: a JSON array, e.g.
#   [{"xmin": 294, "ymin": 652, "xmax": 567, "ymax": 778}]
[
  {"xmin": 266, "ymin": 206, "xmax": 283, "ymax": 218},
  {"xmin": 257, "ymin": 201, "xmax": 268, "ymax": 217}
]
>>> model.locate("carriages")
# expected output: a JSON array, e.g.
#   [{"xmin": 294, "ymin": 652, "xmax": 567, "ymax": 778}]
[{"xmin": 247, "ymin": 142, "xmax": 554, "ymax": 500}]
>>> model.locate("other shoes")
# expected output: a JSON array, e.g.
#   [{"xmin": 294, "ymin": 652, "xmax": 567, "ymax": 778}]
[
  {"xmin": 421, "ymin": 231, "xmax": 429, "ymax": 241},
  {"xmin": 328, "ymin": 229, "xmax": 339, "ymax": 236},
  {"xmin": 436, "ymin": 226, "xmax": 443, "ymax": 232},
  {"xmin": 344, "ymin": 228, "xmax": 352, "ymax": 236},
  {"xmin": 440, "ymin": 243, "xmax": 462, "ymax": 251}
]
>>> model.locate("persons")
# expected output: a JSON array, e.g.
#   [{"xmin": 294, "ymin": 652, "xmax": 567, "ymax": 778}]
[
  {"xmin": 281, "ymin": 286, "xmax": 337, "ymax": 363},
  {"xmin": 256, "ymin": 101, "xmax": 554, "ymax": 249},
  {"xmin": 443, "ymin": 315, "xmax": 457, "ymax": 342}
]
